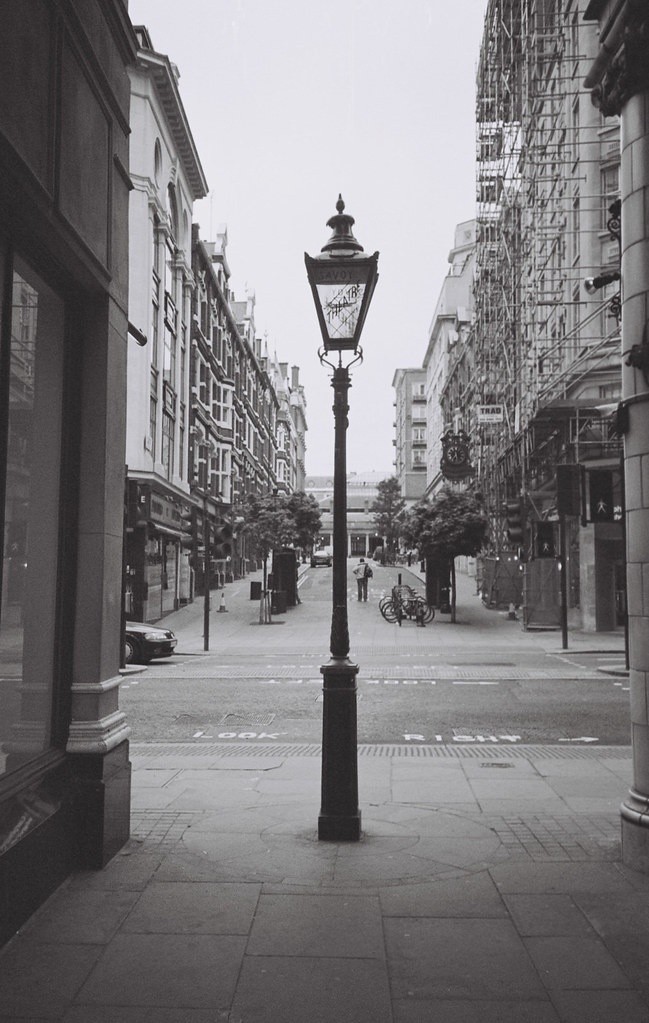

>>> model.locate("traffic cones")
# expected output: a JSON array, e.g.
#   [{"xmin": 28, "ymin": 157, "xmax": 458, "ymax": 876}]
[{"xmin": 217, "ymin": 592, "xmax": 229, "ymax": 613}]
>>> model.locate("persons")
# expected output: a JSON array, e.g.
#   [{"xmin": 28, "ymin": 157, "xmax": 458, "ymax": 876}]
[
  {"xmin": 352, "ymin": 558, "xmax": 368, "ymax": 602},
  {"xmin": 407, "ymin": 552, "xmax": 412, "ymax": 567}
]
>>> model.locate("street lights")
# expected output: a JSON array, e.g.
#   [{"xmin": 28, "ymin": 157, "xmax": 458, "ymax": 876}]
[{"xmin": 303, "ymin": 196, "xmax": 380, "ymax": 839}]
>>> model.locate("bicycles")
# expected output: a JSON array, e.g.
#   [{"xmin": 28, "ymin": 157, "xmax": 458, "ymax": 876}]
[{"xmin": 379, "ymin": 589, "xmax": 435, "ymax": 625}]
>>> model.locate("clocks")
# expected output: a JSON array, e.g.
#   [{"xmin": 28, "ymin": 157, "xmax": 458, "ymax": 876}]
[{"xmin": 446, "ymin": 445, "xmax": 465, "ymax": 465}]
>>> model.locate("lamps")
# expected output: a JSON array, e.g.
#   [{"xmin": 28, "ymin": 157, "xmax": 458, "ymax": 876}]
[{"xmin": 585, "ymin": 196, "xmax": 622, "ymax": 325}]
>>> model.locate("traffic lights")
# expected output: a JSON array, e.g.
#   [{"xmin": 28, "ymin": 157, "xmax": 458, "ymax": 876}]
[
  {"xmin": 213, "ymin": 523, "xmax": 233, "ymax": 559},
  {"xmin": 506, "ymin": 502, "xmax": 527, "ymax": 543},
  {"xmin": 588, "ymin": 470, "xmax": 614, "ymax": 522},
  {"xmin": 556, "ymin": 465, "xmax": 581, "ymax": 514},
  {"xmin": 180, "ymin": 505, "xmax": 198, "ymax": 548},
  {"xmin": 537, "ymin": 521, "xmax": 556, "ymax": 557}
]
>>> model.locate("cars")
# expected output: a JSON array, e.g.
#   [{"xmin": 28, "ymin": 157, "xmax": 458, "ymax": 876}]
[
  {"xmin": 311, "ymin": 551, "xmax": 333, "ymax": 567},
  {"xmin": 124, "ymin": 621, "xmax": 178, "ymax": 664}
]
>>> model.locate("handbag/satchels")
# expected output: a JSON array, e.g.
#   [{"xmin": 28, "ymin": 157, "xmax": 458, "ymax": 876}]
[{"xmin": 364, "ymin": 564, "xmax": 373, "ymax": 577}]
